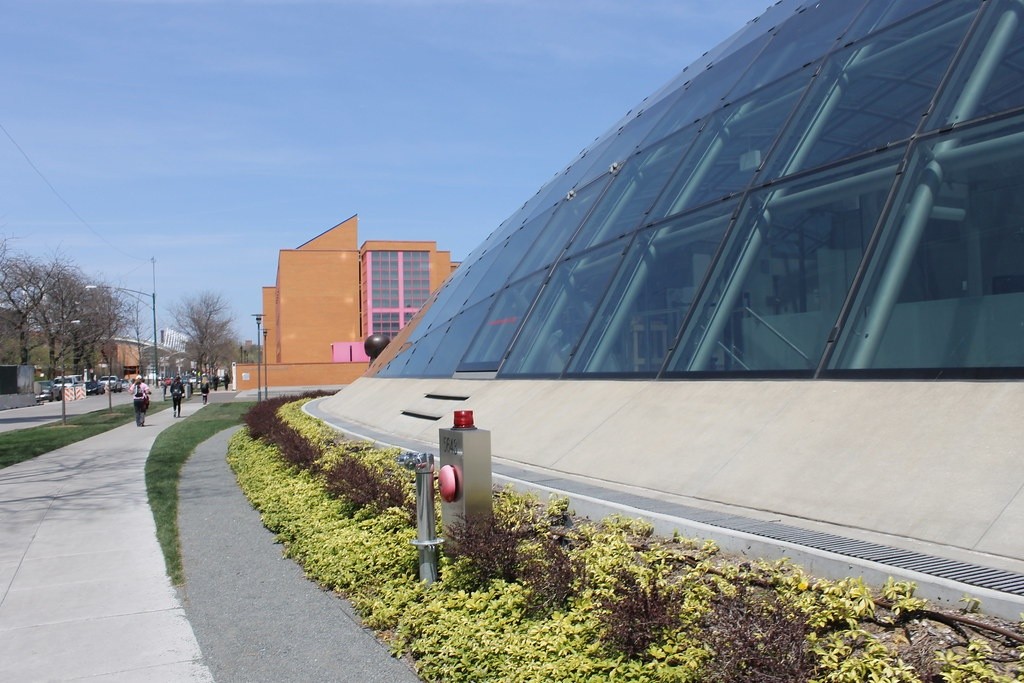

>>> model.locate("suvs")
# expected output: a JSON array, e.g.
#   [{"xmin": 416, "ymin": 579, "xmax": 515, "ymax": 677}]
[{"xmin": 98, "ymin": 374, "xmax": 123, "ymax": 393}]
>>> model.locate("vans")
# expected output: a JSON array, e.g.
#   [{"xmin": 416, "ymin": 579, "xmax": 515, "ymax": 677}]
[{"xmin": 52, "ymin": 374, "xmax": 84, "ymax": 387}]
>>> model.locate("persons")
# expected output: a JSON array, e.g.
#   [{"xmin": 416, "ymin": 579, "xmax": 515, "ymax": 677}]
[
  {"xmin": 169, "ymin": 376, "xmax": 185, "ymax": 418},
  {"xmin": 212, "ymin": 374, "xmax": 219, "ymax": 391},
  {"xmin": 176, "ymin": 373, "xmax": 180, "ymax": 382},
  {"xmin": 160, "ymin": 378, "xmax": 167, "ymax": 402},
  {"xmin": 224, "ymin": 374, "xmax": 230, "ymax": 390},
  {"xmin": 201, "ymin": 376, "xmax": 209, "ymax": 405},
  {"xmin": 128, "ymin": 375, "xmax": 151, "ymax": 427}
]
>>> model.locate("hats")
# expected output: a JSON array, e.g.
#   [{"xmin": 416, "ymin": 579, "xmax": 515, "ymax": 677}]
[{"xmin": 135, "ymin": 375, "xmax": 142, "ymax": 380}]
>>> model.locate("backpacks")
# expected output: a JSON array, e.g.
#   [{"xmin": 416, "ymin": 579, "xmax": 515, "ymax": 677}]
[
  {"xmin": 172, "ymin": 383, "xmax": 181, "ymax": 396},
  {"xmin": 134, "ymin": 382, "xmax": 144, "ymax": 397}
]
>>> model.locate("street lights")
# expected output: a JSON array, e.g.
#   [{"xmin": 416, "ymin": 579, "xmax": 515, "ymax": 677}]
[
  {"xmin": 252, "ymin": 314, "xmax": 262, "ymax": 405},
  {"xmin": 85, "ymin": 285, "xmax": 159, "ymax": 387},
  {"xmin": 23, "ymin": 319, "xmax": 81, "ymax": 379},
  {"xmin": 262, "ymin": 328, "xmax": 271, "ymax": 401}
]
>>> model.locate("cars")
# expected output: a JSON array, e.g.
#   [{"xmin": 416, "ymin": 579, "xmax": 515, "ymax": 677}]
[
  {"xmin": 123, "ymin": 379, "xmax": 133, "ymax": 391},
  {"xmin": 35, "ymin": 385, "xmax": 62, "ymax": 402},
  {"xmin": 86, "ymin": 381, "xmax": 105, "ymax": 395},
  {"xmin": 163, "ymin": 376, "xmax": 200, "ymax": 384}
]
